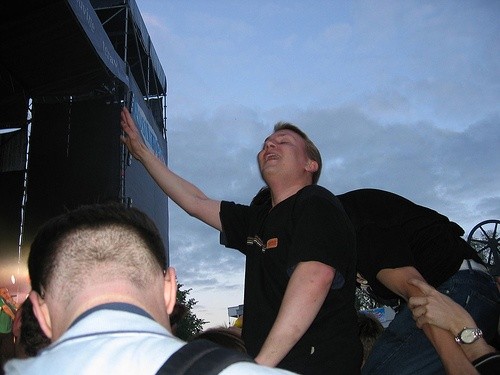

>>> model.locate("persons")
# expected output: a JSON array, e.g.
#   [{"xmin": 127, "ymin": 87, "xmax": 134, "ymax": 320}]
[
  {"xmin": 0, "ymin": 201, "xmax": 500, "ymax": 375},
  {"xmin": 119, "ymin": 106, "xmax": 363, "ymax": 375}
]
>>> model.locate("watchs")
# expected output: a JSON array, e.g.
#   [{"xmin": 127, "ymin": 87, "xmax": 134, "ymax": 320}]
[{"xmin": 453, "ymin": 326, "xmax": 483, "ymax": 345}]
